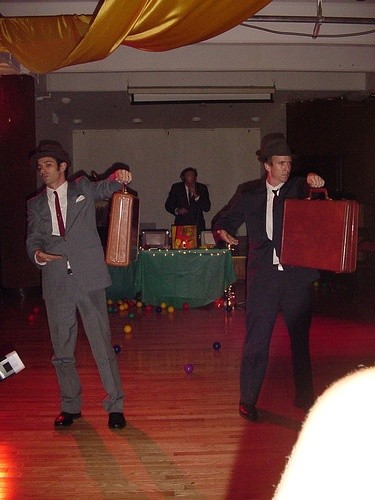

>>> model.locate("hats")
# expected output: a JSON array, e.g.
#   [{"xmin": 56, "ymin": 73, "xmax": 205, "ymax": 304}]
[
  {"xmin": 30, "ymin": 144, "xmax": 70, "ymax": 170},
  {"xmin": 258, "ymin": 137, "xmax": 291, "ymax": 162}
]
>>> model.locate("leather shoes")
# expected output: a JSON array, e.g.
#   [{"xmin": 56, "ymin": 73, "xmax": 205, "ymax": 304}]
[
  {"xmin": 239, "ymin": 402, "xmax": 256, "ymax": 420},
  {"xmin": 54, "ymin": 411, "xmax": 81, "ymax": 427},
  {"xmin": 109, "ymin": 412, "xmax": 125, "ymax": 430}
]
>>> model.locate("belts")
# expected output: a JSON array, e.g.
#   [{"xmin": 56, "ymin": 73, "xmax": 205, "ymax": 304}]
[
  {"xmin": 273, "ymin": 264, "xmax": 284, "ymax": 272},
  {"xmin": 68, "ymin": 269, "xmax": 72, "ymax": 274}
]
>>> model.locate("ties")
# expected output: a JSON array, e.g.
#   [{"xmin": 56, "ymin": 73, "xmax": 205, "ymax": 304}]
[
  {"xmin": 53, "ymin": 191, "xmax": 65, "ymax": 237},
  {"xmin": 272, "ymin": 189, "xmax": 279, "ymax": 257},
  {"xmin": 189, "ymin": 191, "xmax": 191, "ymax": 207}
]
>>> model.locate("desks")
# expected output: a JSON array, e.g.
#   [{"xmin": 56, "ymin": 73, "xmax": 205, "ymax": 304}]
[{"xmin": 139, "ymin": 248, "xmax": 230, "ymax": 311}]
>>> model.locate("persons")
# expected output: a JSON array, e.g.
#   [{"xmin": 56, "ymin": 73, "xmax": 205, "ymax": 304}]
[
  {"xmin": 25, "ymin": 144, "xmax": 133, "ymax": 429},
  {"xmin": 165, "ymin": 167, "xmax": 211, "ymax": 249},
  {"xmin": 210, "ymin": 136, "xmax": 326, "ymax": 421}
]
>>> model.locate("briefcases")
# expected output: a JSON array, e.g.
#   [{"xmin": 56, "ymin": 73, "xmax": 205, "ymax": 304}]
[
  {"xmin": 280, "ymin": 188, "xmax": 360, "ymax": 273},
  {"xmin": 105, "ymin": 182, "xmax": 139, "ymax": 267}
]
